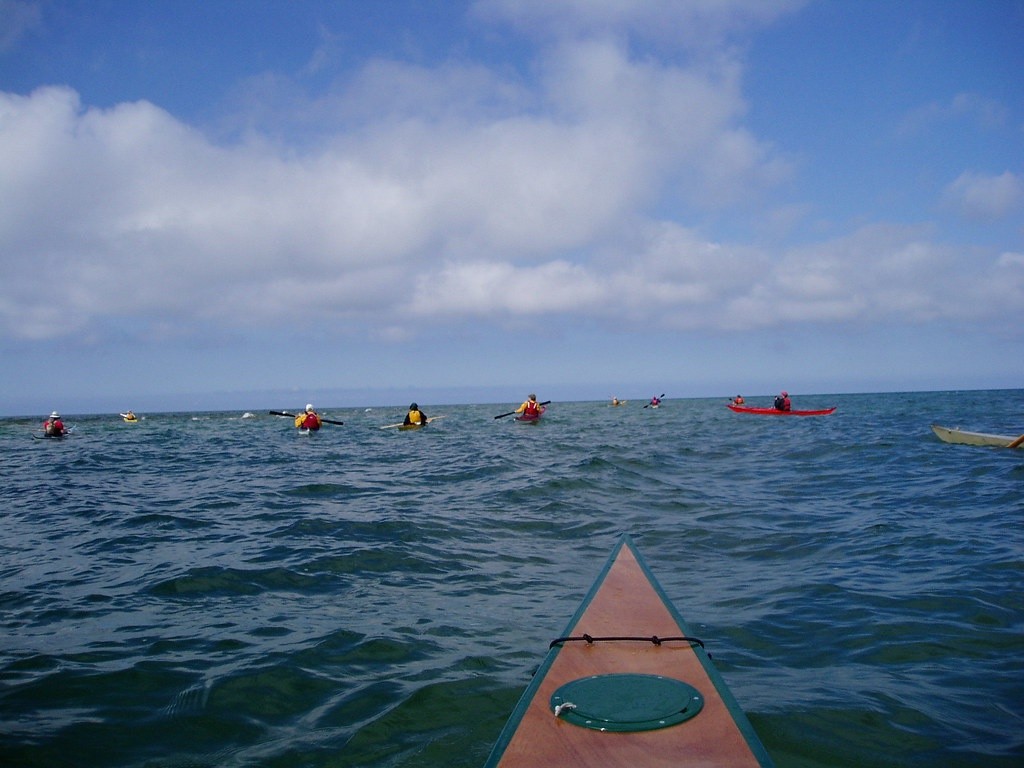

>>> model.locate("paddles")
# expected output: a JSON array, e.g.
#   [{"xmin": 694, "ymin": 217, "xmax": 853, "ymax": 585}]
[
  {"xmin": 379, "ymin": 416, "xmax": 446, "ymax": 430},
  {"xmin": 494, "ymin": 401, "xmax": 551, "ymax": 421},
  {"xmin": 643, "ymin": 393, "xmax": 665, "ymax": 408},
  {"xmin": 269, "ymin": 410, "xmax": 344, "ymax": 426}
]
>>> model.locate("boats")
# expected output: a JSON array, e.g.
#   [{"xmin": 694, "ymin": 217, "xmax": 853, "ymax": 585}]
[
  {"xmin": 32, "ymin": 424, "xmax": 76, "ymax": 441},
  {"xmin": 482, "ymin": 532, "xmax": 773, "ymax": 768},
  {"xmin": 513, "ymin": 415, "xmax": 540, "ymax": 425},
  {"xmin": 298, "ymin": 427, "xmax": 317, "ymax": 436},
  {"xmin": 651, "ymin": 404, "xmax": 660, "ymax": 409},
  {"xmin": 607, "ymin": 403, "xmax": 625, "ymax": 408},
  {"xmin": 929, "ymin": 424, "xmax": 1023, "ymax": 448},
  {"xmin": 123, "ymin": 417, "xmax": 138, "ymax": 422},
  {"xmin": 725, "ymin": 403, "xmax": 836, "ymax": 415},
  {"xmin": 398, "ymin": 425, "xmax": 426, "ymax": 430}
]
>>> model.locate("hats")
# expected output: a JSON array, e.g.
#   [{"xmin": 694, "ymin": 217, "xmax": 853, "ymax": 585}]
[
  {"xmin": 412, "ymin": 403, "xmax": 418, "ymax": 407},
  {"xmin": 50, "ymin": 411, "xmax": 60, "ymax": 418},
  {"xmin": 305, "ymin": 404, "xmax": 313, "ymax": 410},
  {"xmin": 528, "ymin": 394, "xmax": 536, "ymax": 400}
]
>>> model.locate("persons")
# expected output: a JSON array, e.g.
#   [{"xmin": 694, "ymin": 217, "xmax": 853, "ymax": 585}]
[
  {"xmin": 128, "ymin": 411, "xmax": 134, "ymax": 420},
  {"xmin": 735, "ymin": 395, "xmax": 743, "ymax": 404},
  {"xmin": 403, "ymin": 403, "xmax": 427, "ymax": 426},
  {"xmin": 612, "ymin": 397, "xmax": 619, "ymax": 405},
  {"xmin": 44, "ymin": 411, "xmax": 63, "ymax": 437},
  {"xmin": 514, "ymin": 394, "xmax": 546, "ymax": 418},
  {"xmin": 295, "ymin": 404, "xmax": 322, "ymax": 430},
  {"xmin": 774, "ymin": 392, "xmax": 790, "ymax": 411},
  {"xmin": 653, "ymin": 397, "xmax": 657, "ymax": 405}
]
